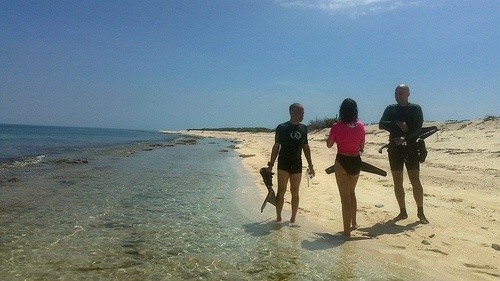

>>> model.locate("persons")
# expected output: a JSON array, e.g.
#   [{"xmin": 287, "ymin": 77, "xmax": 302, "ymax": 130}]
[
  {"xmin": 379, "ymin": 84, "xmax": 430, "ymax": 224},
  {"xmin": 269, "ymin": 102, "xmax": 315, "ymax": 222},
  {"xmin": 327, "ymin": 98, "xmax": 365, "ymax": 237}
]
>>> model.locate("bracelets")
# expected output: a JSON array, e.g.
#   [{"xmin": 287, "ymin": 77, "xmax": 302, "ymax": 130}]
[
  {"xmin": 268, "ymin": 162, "xmax": 274, "ymax": 165},
  {"xmin": 401, "ymin": 137, "xmax": 404, "ymax": 143}
]
